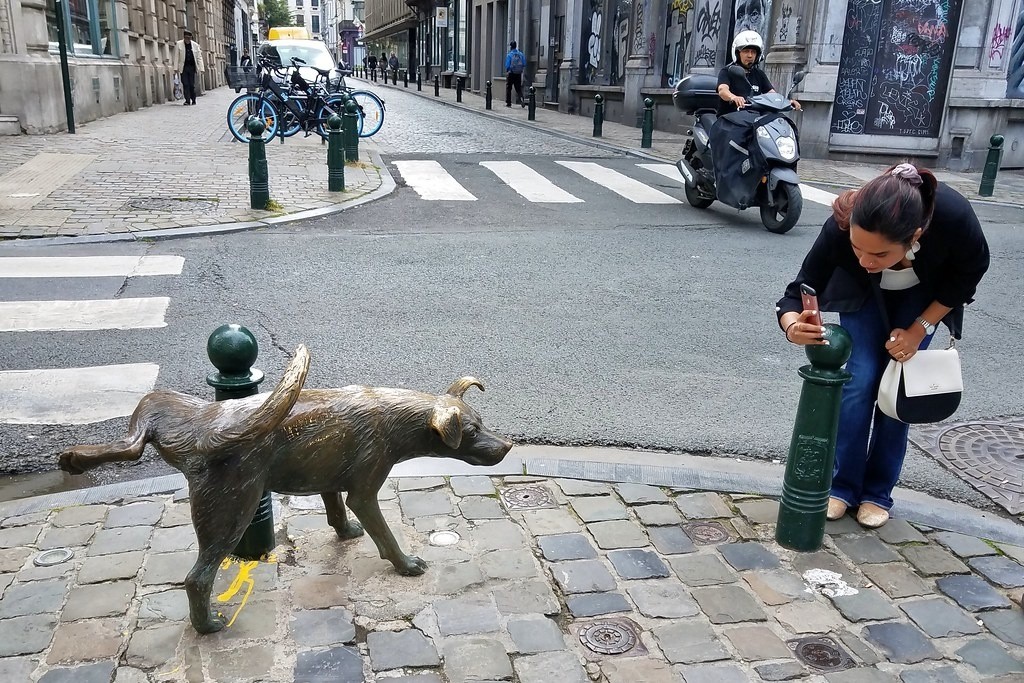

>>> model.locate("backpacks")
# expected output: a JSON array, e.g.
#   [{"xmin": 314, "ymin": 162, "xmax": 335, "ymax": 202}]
[{"xmin": 510, "ymin": 52, "xmax": 524, "ymax": 73}]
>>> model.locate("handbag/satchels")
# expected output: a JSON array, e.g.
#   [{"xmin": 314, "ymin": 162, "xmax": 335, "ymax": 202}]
[
  {"xmin": 173, "ymin": 71, "xmax": 184, "ymax": 100},
  {"xmin": 878, "ymin": 345, "xmax": 963, "ymax": 424}
]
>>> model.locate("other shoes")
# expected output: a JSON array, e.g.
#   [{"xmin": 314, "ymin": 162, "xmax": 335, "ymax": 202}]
[
  {"xmin": 521, "ymin": 96, "xmax": 525, "ymax": 109},
  {"xmin": 192, "ymin": 98, "xmax": 196, "ymax": 105},
  {"xmin": 826, "ymin": 497, "xmax": 848, "ymax": 521},
  {"xmin": 506, "ymin": 103, "xmax": 511, "ymax": 107},
  {"xmin": 856, "ymin": 503, "xmax": 889, "ymax": 528},
  {"xmin": 184, "ymin": 101, "xmax": 190, "ymax": 105}
]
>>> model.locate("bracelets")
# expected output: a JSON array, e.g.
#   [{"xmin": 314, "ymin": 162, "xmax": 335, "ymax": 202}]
[{"xmin": 785, "ymin": 321, "xmax": 796, "ymax": 343}]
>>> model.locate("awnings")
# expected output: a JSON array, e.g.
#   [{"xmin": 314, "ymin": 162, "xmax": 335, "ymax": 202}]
[{"xmin": 405, "ymin": 0, "xmax": 450, "ymax": 20}]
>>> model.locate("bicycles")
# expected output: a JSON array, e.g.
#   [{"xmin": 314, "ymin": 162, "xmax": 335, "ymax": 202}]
[{"xmin": 224, "ymin": 52, "xmax": 386, "ymax": 142}]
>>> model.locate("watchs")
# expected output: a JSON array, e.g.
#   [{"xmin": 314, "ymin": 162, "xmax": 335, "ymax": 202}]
[{"xmin": 915, "ymin": 315, "xmax": 936, "ymax": 335}]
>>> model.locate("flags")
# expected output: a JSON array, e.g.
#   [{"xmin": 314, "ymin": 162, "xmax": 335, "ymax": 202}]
[{"xmin": 352, "ymin": 17, "xmax": 362, "ymax": 28}]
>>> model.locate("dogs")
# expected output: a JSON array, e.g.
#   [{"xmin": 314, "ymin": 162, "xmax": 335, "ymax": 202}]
[{"xmin": 58, "ymin": 344, "xmax": 519, "ymax": 631}]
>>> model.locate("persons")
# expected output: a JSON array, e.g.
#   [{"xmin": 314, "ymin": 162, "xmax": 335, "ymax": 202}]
[
  {"xmin": 174, "ymin": 30, "xmax": 205, "ymax": 105},
  {"xmin": 379, "ymin": 53, "xmax": 388, "ymax": 80},
  {"xmin": 505, "ymin": 41, "xmax": 527, "ymax": 108},
  {"xmin": 715, "ymin": 29, "xmax": 801, "ymax": 187},
  {"xmin": 240, "ymin": 48, "xmax": 253, "ymax": 80},
  {"xmin": 775, "ymin": 164, "xmax": 990, "ymax": 528},
  {"xmin": 425, "ymin": 55, "xmax": 433, "ymax": 86},
  {"xmin": 363, "ymin": 52, "xmax": 377, "ymax": 73},
  {"xmin": 389, "ymin": 52, "xmax": 400, "ymax": 86}
]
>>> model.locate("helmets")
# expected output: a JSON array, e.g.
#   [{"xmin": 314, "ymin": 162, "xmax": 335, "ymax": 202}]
[{"xmin": 731, "ymin": 30, "xmax": 766, "ymax": 66}]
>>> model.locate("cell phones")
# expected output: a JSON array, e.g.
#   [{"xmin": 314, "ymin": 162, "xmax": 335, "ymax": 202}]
[{"xmin": 800, "ymin": 283, "xmax": 822, "ymax": 334}]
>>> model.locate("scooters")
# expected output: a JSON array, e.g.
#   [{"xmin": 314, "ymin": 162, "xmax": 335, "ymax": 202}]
[{"xmin": 673, "ymin": 71, "xmax": 804, "ymax": 234}]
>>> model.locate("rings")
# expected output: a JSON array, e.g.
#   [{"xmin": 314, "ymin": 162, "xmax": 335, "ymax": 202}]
[{"xmin": 900, "ymin": 350, "xmax": 905, "ymax": 357}]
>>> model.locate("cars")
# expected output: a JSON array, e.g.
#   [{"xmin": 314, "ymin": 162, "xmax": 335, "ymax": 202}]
[
  {"xmin": 244, "ymin": 27, "xmax": 347, "ymax": 112},
  {"xmin": 449, "ymin": 51, "xmax": 466, "ymax": 70}
]
